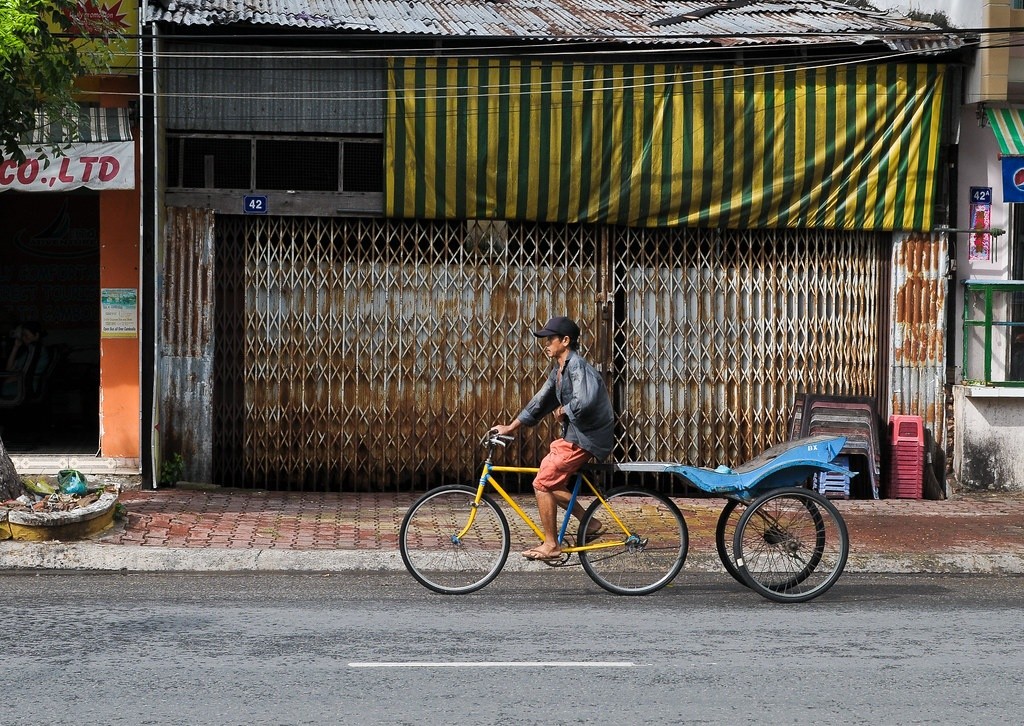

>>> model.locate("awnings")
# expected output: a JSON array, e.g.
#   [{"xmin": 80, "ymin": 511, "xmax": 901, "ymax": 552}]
[
  {"xmin": 985, "ymin": 107, "xmax": 1024, "ymax": 203},
  {"xmin": 0, "ymin": 107, "xmax": 135, "ymax": 191}
]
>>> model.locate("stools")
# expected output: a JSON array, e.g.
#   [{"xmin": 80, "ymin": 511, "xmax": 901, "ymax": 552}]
[{"xmin": 786, "ymin": 393, "xmax": 925, "ymax": 499}]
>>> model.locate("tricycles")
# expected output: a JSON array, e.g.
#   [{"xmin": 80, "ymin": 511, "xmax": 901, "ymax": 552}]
[{"xmin": 398, "ymin": 429, "xmax": 860, "ymax": 605}]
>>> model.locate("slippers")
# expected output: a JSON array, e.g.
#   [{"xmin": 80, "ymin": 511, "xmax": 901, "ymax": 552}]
[
  {"xmin": 584, "ymin": 527, "xmax": 609, "ymax": 544},
  {"xmin": 522, "ymin": 548, "xmax": 564, "ymax": 561}
]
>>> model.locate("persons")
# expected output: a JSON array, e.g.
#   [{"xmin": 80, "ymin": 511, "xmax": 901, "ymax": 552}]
[
  {"xmin": 491, "ymin": 317, "xmax": 615, "ymax": 560},
  {"xmin": 0, "ymin": 324, "xmax": 50, "ymax": 437}
]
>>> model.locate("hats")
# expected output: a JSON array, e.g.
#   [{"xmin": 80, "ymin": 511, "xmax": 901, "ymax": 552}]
[{"xmin": 532, "ymin": 316, "xmax": 581, "ymax": 340}]
